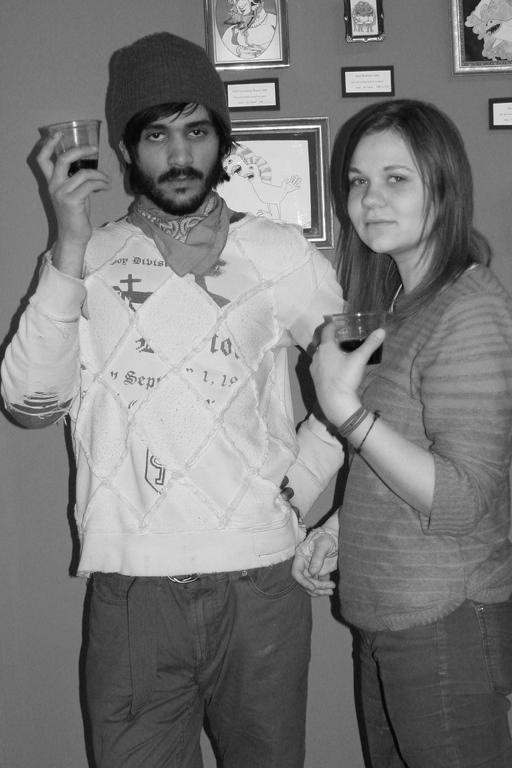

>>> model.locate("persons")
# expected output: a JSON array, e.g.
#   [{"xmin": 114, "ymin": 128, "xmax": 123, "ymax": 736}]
[
  {"xmin": 295, "ymin": 100, "xmax": 512, "ymax": 766},
  {"xmin": 0, "ymin": 31, "xmax": 348, "ymax": 766}
]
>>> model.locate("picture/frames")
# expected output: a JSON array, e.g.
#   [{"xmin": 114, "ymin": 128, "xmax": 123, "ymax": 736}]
[
  {"xmin": 221, "ymin": 117, "xmax": 334, "ymax": 251},
  {"xmin": 204, "ymin": 0, "xmax": 291, "ymax": 70},
  {"xmin": 223, "ymin": 78, "xmax": 280, "ymax": 110},
  {"xmin": 488, "ymin": 97, "xmax": 512, "ymax": 130},
  {"xmin": 450, "ymin": 0, "xmax": 512, "ymax": 76},
  {"xmin": 342, "ymin": 67, "xmax": 395, "ymax": 97},
  {"xmin": 344, "ymin": 0, "xmax": 386, "ymax": 44}
]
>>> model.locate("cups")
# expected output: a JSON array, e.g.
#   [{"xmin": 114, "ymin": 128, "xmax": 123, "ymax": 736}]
[
  {"xmin": 47, "ymin": 119, "xmax": 102, "ymax": 177},
  {"xmin": 331, "ymin": 310, "xmax": 384, "ymax": 365}
]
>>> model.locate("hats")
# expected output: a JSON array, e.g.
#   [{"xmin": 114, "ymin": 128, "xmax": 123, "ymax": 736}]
[{"xmin": 107, "ymin": 27, "xmax": 233, "ymax": 167}]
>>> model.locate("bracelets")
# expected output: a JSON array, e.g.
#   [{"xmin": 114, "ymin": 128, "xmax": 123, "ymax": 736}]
[{"xmin": 328, "ymin": 405, "xmax": 382, "ymax": 452}]
